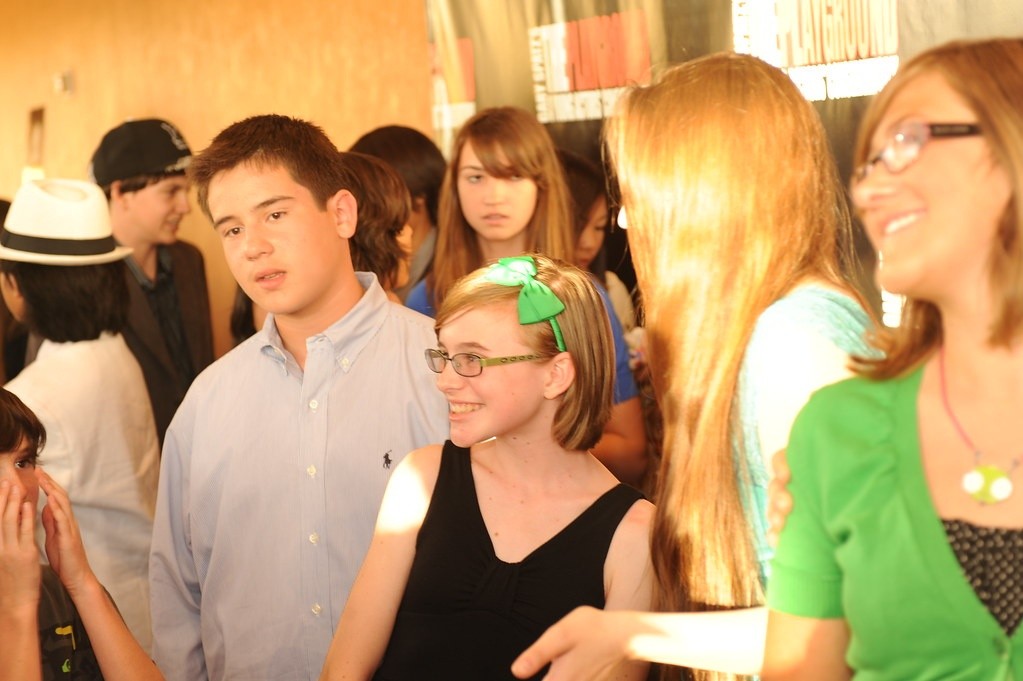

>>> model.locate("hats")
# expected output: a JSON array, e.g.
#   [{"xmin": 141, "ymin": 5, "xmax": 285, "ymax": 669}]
[
  {"xmin": 0, "ymin": 168, "xmax": 133, "ymax": 264},
  {"xmin": 90, "ymin": 116, "xmax": 194, "ymax": 184}
]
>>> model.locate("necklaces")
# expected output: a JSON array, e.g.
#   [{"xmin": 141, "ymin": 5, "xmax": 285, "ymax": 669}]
[{"xmin": 938, "ymin": 346, "xmax": 1023, "ymax": 507}]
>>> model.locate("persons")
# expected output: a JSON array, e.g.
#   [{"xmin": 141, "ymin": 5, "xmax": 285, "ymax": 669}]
[
  {"xmin": 145, "ymin": 117, "xmax": 452, "ymax": 680},
  {"xmin": 91, "ymin": 118, "xmax": 217, "ymax": 452},
  {"xmin": 553, "ymin": 145, "xmax": 658, "ymax": 416},
  {"xmin": 334, "ymin": 152, "xmax": 415, "ymax": 306},
  {"xmin": 405, "ymin": 106, "xmax": 646, "ymax": 477},
  {"xmin": 1, "ymin": 387, "xmax": 166, "ymax": 681},
  {"xmin": 348, "ymin": 125, "xmax": 450, "ymax": 306},
  {"xmin": 762, "ymin": 39, "xmax": 1023, "ymax": 680},
  {"xmin": 767, "ymin": 448, "xmax": 793, "ymax": 534},
  {"xmin": 318, "ymin": 251, "xmax": 669, "ymax": 680},
  {"xmin": 510, "ymin": 50, "xmax": 890, "ymax": 681},
  {"xmin": 1, "ymin": 179, "xmax": 161, "ymax": 667}
]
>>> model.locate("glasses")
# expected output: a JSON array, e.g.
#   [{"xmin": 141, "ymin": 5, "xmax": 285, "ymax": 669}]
[
  {"xmin": 423, "ymin": 345, "xmax": 551, "ymax": 378},
  {"xmin": 848, "ymin": 120, "xmax": 983, "ymax": 199}
]
